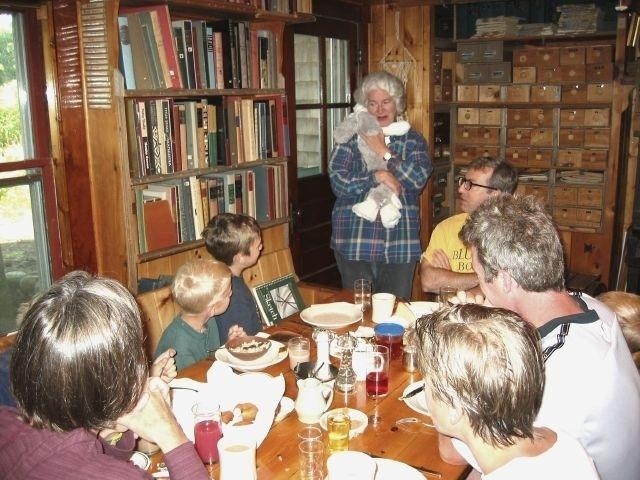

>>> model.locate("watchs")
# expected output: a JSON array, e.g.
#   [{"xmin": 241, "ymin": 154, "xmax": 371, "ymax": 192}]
[{"xmin": 382, "ymin": 151, "xmax": 397, "ymax": 161}]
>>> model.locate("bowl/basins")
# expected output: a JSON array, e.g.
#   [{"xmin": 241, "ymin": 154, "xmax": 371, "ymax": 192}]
[
  {"xmin": 327, "ymin": 449, "xmax": 375, "ymax": 480},
  {"xmin": 225, "ymin": 337, "xmax": 270, "ymax": 362},
  {"xmin": 375, "ymin": 324, "xmax": 403, "ymax": 346}
]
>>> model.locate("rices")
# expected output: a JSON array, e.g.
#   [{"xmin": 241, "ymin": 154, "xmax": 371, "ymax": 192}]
[{"xmin": 229, "ymin": 340, "xmax": 271, "ymax": 354}]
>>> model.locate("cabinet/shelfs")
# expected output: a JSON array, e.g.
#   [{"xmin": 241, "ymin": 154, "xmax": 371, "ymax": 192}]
[
  {"xmin": 79, "ymin": 0, "xmax": 300, "ymax": 360},
  {"xmin": 455, "ymin": 29, "xmax": 640, "ymax": 293},
  {"xmin": 368, "ymin": 0, "xmax": 458, "ymax": 256}
]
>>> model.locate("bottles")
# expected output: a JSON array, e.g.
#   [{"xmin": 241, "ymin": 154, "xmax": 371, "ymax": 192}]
[{"xmin": 310, "ymin": 327, "xmax": 356, "ymax": 395}]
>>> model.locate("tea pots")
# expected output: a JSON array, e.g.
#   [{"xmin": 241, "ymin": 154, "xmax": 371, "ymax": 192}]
[{"xmin": 295, "ymin": 378, "xmax": 334, "ymax": 425}]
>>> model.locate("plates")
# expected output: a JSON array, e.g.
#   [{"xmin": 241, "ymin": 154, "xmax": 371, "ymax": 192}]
[
  {"xmin": 294, "ymin": 361, "xmax": 340, "ymax": 383},
  {"xmin": 320, "ymin": 407, "xmax": 369, "ymax": 437},
  {"xmin": 275, "ymin": 397, "xmax": 294, "ymax": 422},
  {"xmin": 215, "ymin": 340, "xmax": 288, "ymax": 373},
  {"xmin": 301, "ymin": 301, "xmax": 364, "ymax": 330},
  {"xmin": 405, "ymin": 381, "xmax": 431, "ymax": 415},
  {"xmin": 371, "ymin": 456, "xmax": 425, "ymax": 480},
  {"xmin": 400, "ymin": 300, "xmax": 438, "ymax": 329},
  {"xmin": 131, "ymin": 451, "xmax": 151, "ymax": 471}
]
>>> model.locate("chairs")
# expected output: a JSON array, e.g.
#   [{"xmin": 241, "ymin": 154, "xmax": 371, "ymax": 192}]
[
  {"xmin": 409, "ymin": 261, "xmax": 436, "ymax": 301},
  {"xmin": 238, "ymin": 248, "xmax": 336, "ymax": 327},
  {"xmin": 136, "ymin": 283, "xmax": 183, "ymax": 360}
]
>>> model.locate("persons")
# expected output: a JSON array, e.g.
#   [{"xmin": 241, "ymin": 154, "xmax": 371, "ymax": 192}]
[
  {"xmin": 414, "ymin": 302, "xmax": 601, "ymax": 480},
  {"xmin": 153, "ymin": 258, "xmax": 234, "ymax": 371},
  {"xmin": 202, "ymin": 212, "xmax": 264, "ymax": 346},
  {"xmin": 458, "ymin": 192, "xmax": 640, "ymax": 480},
  {"xmin": 417, "ymin": 155, "xmax": 519, "ymax": 302},
  {"xmin": 326, "ymin": 69, "xmax": 434, "ymax": 302},
  {"xmin": 1, "ymin": 270, "xmax": 210, "ymax": 480}
]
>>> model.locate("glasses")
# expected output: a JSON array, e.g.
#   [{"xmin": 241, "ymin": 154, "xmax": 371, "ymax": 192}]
[{"xmin": 458, "ymin": 177, "xmax": 497, "ymax": 191}]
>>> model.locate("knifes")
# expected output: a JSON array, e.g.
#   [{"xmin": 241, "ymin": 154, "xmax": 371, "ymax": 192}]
[{"xmin": 399, "ymin": 385, "xmax": 425, "ymax": 401}]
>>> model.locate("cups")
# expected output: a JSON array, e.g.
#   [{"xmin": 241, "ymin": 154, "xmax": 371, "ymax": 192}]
[
  {"xmin": 295, "ymin": 412, "xmax": 349, "ymax": 479},
  {"xmin": 353, "ymin": 350, "xmax": 384, "ymax": 380},
  {"xmin": 218, "ymin": 434, "xmax": 257, "ymax": 480},
  {"xmin": 439, "ymin": 285, "xmax": 459, "ymax": 306},
  {"xmin": 372, "ymin": 292, "xmax": 396, "ymax": 324},
  {"xmin": 403, "ymin": 346, "xmax": 419, "ymax": 373},
  {"xmin": 191, "ymin": 402, "xmax": 222, "ymax": 463},
  {"xmin": 354, "ymin": 277, "xmax": 372, "ymax": 308},
  {"xmin": 364, "ymin": 344, "xmax": 390, "ymax": 399},
  {"xmin": 288, "ymin": 336, "xmax": 311, "ymax": 371}
]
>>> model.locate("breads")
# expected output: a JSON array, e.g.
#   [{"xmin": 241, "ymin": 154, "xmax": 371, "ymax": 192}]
[{"xmin": 221, "ymin": 403, "xmax": 258, "ymax": 426}]
